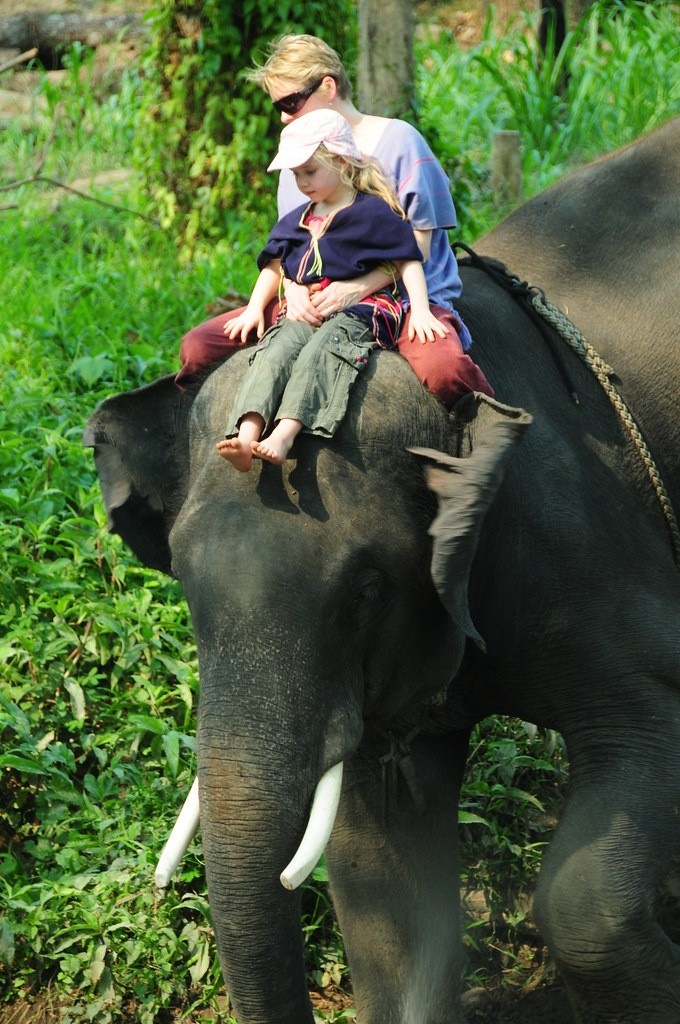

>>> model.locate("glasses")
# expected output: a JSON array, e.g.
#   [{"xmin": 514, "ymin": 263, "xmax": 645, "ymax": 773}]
[{"xmin": 272, "ymin": 75, "xmax": 339, "ymax": 115}]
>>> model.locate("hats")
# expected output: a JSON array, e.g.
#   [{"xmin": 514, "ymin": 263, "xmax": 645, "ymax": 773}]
[{"xmin": 267, "ymin": 110, "xmax": 363, "ymax": 172}]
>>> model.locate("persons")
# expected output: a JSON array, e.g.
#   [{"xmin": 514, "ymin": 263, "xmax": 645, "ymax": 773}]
[
  {"xmin": 216, "ymin": 108, "xmax": 451, "ymax": 473},
  {"xmin": 175, "ymin": 35, "xmax": 495, "ymax": 411}
]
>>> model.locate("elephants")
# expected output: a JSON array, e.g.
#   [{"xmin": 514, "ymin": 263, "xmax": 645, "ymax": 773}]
[{"xmin": 82, "ymin": 115, "xmax": 680, "ymax": 1023}]
